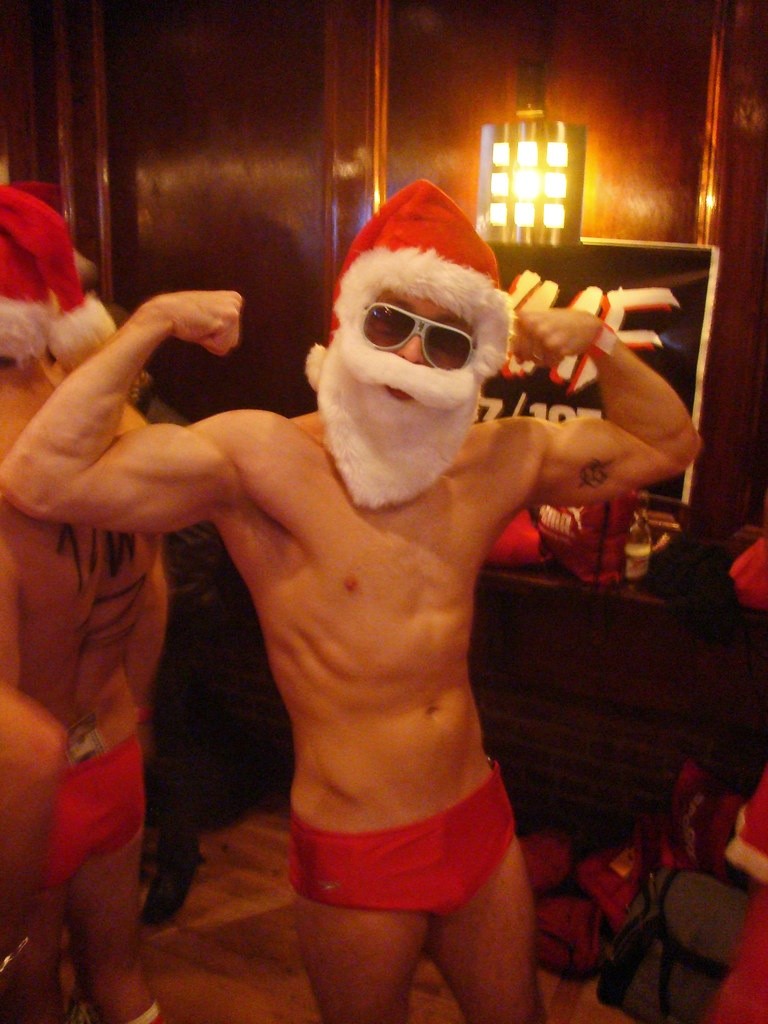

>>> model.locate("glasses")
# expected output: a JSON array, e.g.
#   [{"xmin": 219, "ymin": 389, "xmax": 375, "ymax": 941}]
[{"xmin": 362, "ymin": 303, "xmax": 473, "ymax": 371}]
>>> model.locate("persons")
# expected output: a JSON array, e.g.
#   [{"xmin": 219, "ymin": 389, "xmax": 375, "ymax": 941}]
[
  {"xmin": 0, "ymin": 184, "xmax": 168, "ymax": 1024},
  {"xmin": 2, "ymin": 180, "xmax": 702, "ymax": 1024}
]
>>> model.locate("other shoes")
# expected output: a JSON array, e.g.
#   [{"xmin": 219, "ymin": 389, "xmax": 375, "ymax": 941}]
[{"xmin": 141, "ymin": 848, "xmax": 199, "ymax": 927}]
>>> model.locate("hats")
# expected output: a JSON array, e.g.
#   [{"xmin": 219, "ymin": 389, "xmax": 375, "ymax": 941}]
[
  {"xmin": 0, "ymin": 185, "xmax": 116, "ymax": 368},
  {"xmin": 304, "ymin": 179, "xmax": 515, "ymax": 378}
]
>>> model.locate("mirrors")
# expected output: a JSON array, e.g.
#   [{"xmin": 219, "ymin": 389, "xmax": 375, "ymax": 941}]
[{"xmin": 471, "ymin": 241, "xmax": 724, "ymax": 508}]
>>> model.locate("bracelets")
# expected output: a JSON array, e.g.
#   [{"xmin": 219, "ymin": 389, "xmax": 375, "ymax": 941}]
[{"xmin": 588, "ymin": 324, "xmax": 616, "ymax": 363}]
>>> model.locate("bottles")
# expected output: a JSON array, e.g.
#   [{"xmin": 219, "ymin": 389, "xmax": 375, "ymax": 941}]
[{"xmin": 624, "ymin": 517, "xmax": 651, "ymax": 580}]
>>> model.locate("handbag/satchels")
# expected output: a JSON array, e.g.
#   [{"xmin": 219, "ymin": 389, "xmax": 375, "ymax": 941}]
[{"xmin": 535, "ymin": 483, "xmax": 636, "ymax": 593}]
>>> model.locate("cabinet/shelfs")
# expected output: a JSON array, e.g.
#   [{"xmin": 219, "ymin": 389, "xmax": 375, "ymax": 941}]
[{"xmin": 165, "ymin": 531, "xmax": 767, "ymax": 847}]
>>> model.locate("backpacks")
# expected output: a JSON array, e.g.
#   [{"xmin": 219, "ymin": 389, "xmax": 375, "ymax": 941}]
[{"xmin": 536, "ymin": 813, "xmax": 750, "ymax": 1024}]
